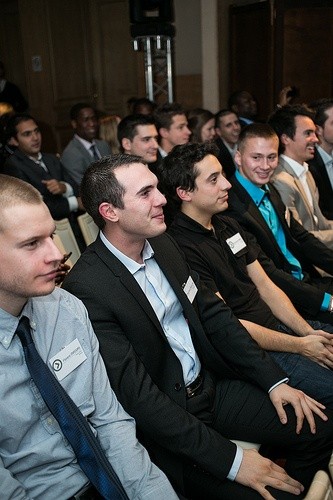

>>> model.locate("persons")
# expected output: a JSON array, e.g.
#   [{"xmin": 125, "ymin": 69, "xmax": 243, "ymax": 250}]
[
  {"xmin": 0, "ymin": 173, "xmax": 183, "ymax": 500},
  {"xmin": 131, "ymin": 96, "xmax": 156, "ymax": 120},
  {"xmin": 268, "ymin": 102, "xmax": 333, "ymax": 250},
  {"xmin": 159, "ymin": 138, "xmax": 333, "ymax": 419},
  {"xmin": 187, "ymin": 108, "xmax": 216, "ymax": 145},
  {"xmin": 60, "ymin": 154, "xmax": 333, "ymax": 500},
  {"xmin": 212, "ymin": 107, "xmax": 244, "ymax": 180},
  {"xmin": 0, "ymin": 110, "xmax": 89, "ymax": 256},
  {"xmin": 222, "ymin": 120, "xmax": 333, "ymax": 324},
  {"xmin": 97, "ymin": 114, "xmax": 124, "ymax": 157},
  {"xmin": 148, "ymin": 103, "xmax": 191, "ymax": 171},
  {"xmin": 226, "ymin": 86, "xmax": 292, "ymax": 132},
  {"xmin": 0, "ymin": 62, "xmax": 28, "ymax": 114},
  {"xmin": 59, "ymin": 102, "xmax": 114, "ymax": 188},
  {"xmin": 313, "ymin": 98, "xmax": 333, "ymax": 221},
  {"xmin": 117, "ymin": 112, "xmax": 159, "ymax": 166}
]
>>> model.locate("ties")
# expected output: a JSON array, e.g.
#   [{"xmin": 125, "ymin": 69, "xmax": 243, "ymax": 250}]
[
  {"xmin": 17, "ymin": 316, "xmax": 129, "ymax": 500},
  {"xmin": 90, "ymin": 145, "xmax": 100, "ymax": 161}
]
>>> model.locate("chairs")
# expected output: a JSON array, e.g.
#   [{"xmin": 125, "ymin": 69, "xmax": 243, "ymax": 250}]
[
  {"xmin": 77, "ymin": 212, "xmax": 102, "ymax": 247},
  {"xmin": 51, "ymin": 217, "xmax": 82, "ymax": 288}
]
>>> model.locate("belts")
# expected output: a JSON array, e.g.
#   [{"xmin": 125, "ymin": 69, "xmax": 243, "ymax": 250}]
[
  {"xmin": 184, "ymin": 364, "xmax": 206, "ymax": 399},
  {"xmin": 70, "ymin": 483, "xmax": 100, "ymax": 500}
]
